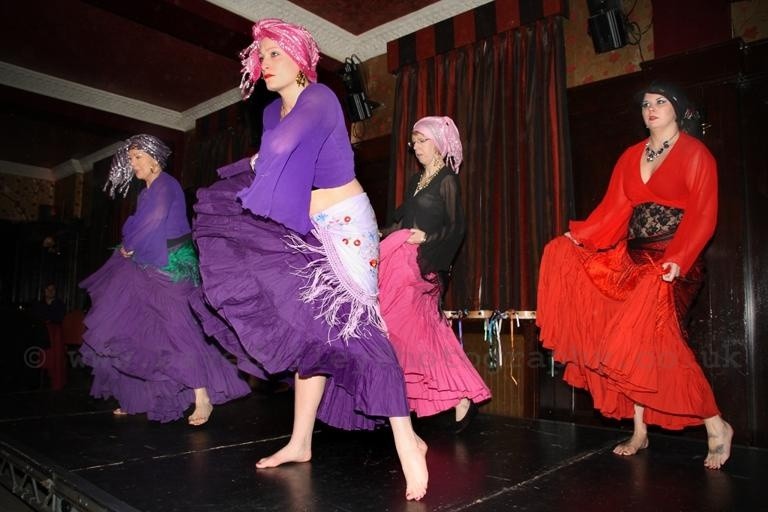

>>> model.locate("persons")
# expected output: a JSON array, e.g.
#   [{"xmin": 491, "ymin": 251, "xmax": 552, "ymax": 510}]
[
  {"xmin": 187, "ymin": 17, "xmax": 430, "ymax": 501},
  {"xmin": 536, "ymin": 82, "xmax": 735, "ymax": 470},
  {"xmin": 380, "ymin": 116, "xmax": 493, "ymax": 435},
  {"xmin": 34, "ymin": 282, "xmax": 66, "ymax": 325},
  {"xmin": 78, "ymin": 133, "xmax": 253, "ymax": 426}
]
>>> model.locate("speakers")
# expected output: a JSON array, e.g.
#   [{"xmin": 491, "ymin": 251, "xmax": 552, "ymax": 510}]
[
  {"xmin": 587, "ymin": 9, "xmax": 630, "ymax": 54},
  {"xmin": 335, "ymin": 78, "xmax": 373, "ymax": 121}
]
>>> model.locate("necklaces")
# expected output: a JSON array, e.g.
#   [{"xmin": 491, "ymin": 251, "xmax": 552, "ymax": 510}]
[
  {"xmin": 282, "ymin": 104, "xmax": 287, "ymax": 119},
  {"xmin": 644, "ymin": 131, "xmax": 682, "ymax": 162},
  {"xmin": 417, "ymin": 165, "xmax": 446, "ymax": 191}
]
